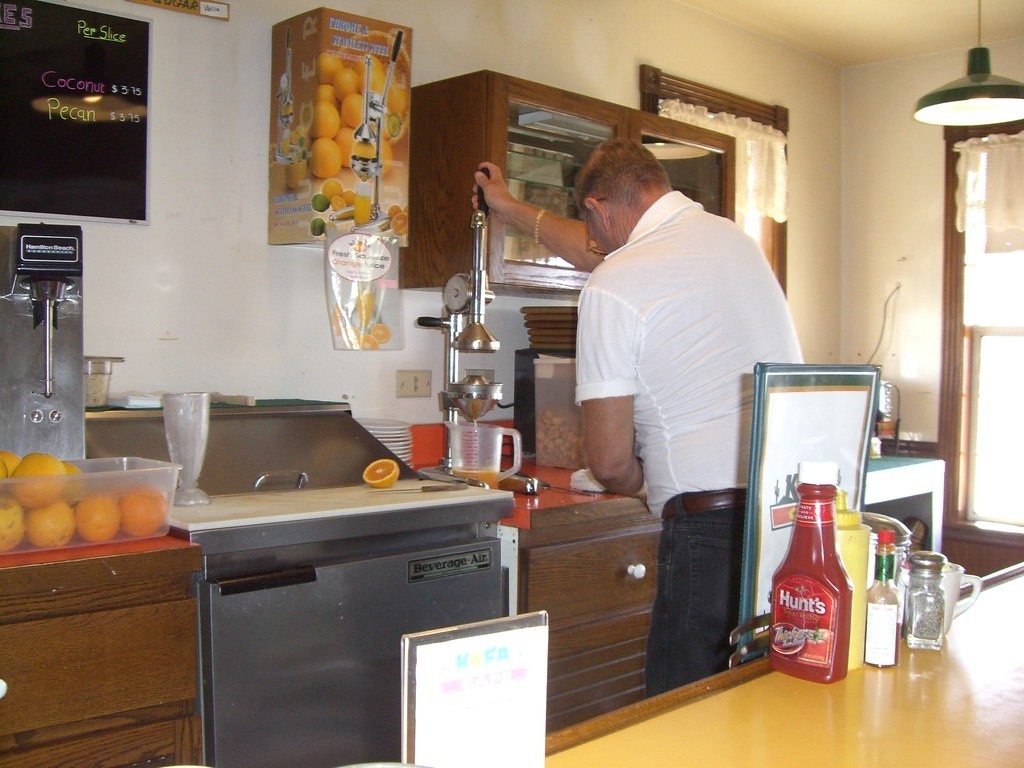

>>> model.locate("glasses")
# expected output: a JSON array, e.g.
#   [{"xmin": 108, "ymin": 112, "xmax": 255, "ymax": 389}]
[{"xmin": 585, "ymin": 198, "xmax": 609, "ymax": 256}]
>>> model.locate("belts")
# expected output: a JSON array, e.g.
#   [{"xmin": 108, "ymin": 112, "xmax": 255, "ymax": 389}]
[{"xmin": 661, "ymin": 491, "xmax": 745, "ymax": 519}]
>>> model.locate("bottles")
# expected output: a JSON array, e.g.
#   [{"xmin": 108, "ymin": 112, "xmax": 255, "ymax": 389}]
[{"xmin": 767, "ymin": 463, "xmax": 948, "ymax": 684}]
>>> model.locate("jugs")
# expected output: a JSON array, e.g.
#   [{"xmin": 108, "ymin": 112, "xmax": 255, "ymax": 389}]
[{"xmin": 443, "ymin": 420, "xmax": 521, "ymax": 490}]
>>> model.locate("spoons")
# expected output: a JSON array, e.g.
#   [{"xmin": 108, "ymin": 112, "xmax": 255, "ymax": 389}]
[{"xmin": 538, "ymin": 479, "xmax": 595, "ymax": 497}]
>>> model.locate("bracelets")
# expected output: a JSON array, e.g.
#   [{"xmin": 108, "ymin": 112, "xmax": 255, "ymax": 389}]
[{"xmin": 534, "ymin": 209, "xmax": 548, "ymax": 247}]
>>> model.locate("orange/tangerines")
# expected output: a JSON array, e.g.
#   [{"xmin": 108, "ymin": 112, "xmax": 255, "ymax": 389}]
[
  {"xmin": 362, "ymin": 459, "xmax": 400, "ymax": 488},
  {"xmin": 0, "ymin": 449, "xmax": 169, "ymax": 552},
  {"xmin": 331, "ymin": 305, "xmax": 391, "ymax": 350},
  {"xmin": 309, "ymin": 51, "xmax": 408, "ymax": 236}
]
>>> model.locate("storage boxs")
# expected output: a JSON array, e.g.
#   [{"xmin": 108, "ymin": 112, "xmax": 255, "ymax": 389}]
[{"xmin": 0, "ymin": 455, "xmax": 182, "ymax": 562}]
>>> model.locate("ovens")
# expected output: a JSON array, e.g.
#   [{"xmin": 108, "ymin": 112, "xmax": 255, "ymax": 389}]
[{"xmin": 199, "ymin": 537, "xmax": 503, "ymax": 768}]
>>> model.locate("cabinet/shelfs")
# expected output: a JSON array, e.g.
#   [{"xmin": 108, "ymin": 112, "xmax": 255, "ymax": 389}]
[
  {"xmin": 397, "ymin": 68, "xmax": 736, "ymax": 293},
  {"xmin": 520, "ymin": 498, "xmax": 663, "ymax": 734},
  {"xmin": 0, "ymin": 539, "xmax": 205, "ymax": 768}
]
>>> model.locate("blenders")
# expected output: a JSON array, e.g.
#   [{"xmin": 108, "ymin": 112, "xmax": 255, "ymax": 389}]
[
  {"xmin": 418, "ymin": 168, "xmax": 538, "ymax": 495},
  {"xmin": 273, "ymin": 26, "xmax": 299, "ymax": 164},
  {"xmin": 330, "ymin": 30, "xmax": 403, "ymax": 233}
]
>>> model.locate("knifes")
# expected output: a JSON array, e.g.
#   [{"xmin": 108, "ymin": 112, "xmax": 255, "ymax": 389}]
[{"xmin": 367, "ymin": 482, "xmax": 468, "ymax": 493}]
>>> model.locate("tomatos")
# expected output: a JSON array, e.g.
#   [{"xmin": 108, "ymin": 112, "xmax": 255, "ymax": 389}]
[{"xmin": 798, "ymin": 628, "xmax": 834, "ymax": 663}]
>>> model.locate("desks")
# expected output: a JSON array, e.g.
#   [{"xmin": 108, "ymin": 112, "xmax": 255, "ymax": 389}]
[
  {"xmin": 541, "ymin": 561, "xmax": 1024, "ymax": 768},
  {"xmin": 866, "ymin": 457, "xmax": 947, "ymax": 555}
]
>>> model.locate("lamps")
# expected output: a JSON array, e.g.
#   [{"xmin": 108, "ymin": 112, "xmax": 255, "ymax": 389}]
[{"xmin": 912, "ymin": 0, "xmax": 1024, "ymax": 124}]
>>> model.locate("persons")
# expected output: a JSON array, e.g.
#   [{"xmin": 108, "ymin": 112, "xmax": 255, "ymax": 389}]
[{"xmin": 470, "ymin": 136, "xmax": 804, "ymax": 697}]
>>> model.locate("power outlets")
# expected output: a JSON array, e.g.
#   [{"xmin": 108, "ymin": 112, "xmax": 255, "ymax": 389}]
[{"xmin": 396, "ymin": 369, "xmax": 432, "ymax": 398}]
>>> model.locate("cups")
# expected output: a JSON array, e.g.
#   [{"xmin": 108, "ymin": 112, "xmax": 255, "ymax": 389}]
[
  {"xmin": 355, "ymin": 183, "xmax": 371, "ymax": 226},
  {"xmin": 161, "ymin": 392, "xmax": 211, "ymax": 506},
  {"xmin": 900, "ymin": 562, "xmax": 982, "ymax": 637}
]
